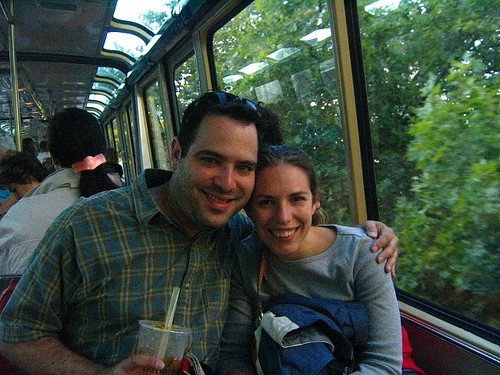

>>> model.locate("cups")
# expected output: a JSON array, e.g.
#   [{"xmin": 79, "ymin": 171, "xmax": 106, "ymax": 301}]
[{"xmin": 132, "ymin": 318, "xmax": 192, "ymax": 374}]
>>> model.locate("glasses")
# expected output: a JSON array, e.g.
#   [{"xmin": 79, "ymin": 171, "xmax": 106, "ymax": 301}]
[{"xmin": 183, "ymin": 93, "xmax": 259, "ymax": 127}]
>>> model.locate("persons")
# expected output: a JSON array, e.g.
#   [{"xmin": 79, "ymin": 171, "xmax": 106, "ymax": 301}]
[
  {"xmin": 219, "ymin": 145, "xmax": 424, "ymax": 375},
  {"xmin": 0, "ymin": 90, "xmax": 401, "ymax": 375},
  {"xmin": 0, "ymin": 106, "xmax": 127, "ymax": 277},
  {"xmin": 0, "ymin": 137, "xmax": 54, "ymax": 220}
]
